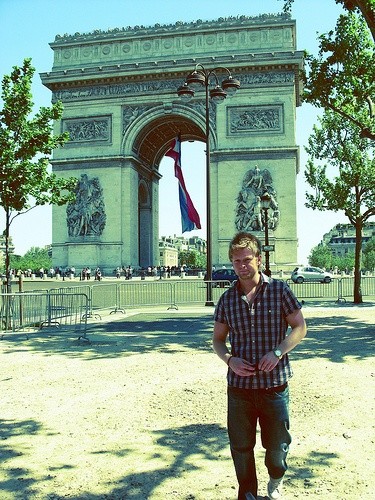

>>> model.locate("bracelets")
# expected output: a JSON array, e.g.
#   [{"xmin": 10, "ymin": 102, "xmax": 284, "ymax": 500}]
[{"xmin": 227, "ymin": 355, "xmax": 233, "ymax": 365}]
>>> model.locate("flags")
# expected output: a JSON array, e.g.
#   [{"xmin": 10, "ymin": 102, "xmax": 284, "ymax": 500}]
[{"xmin": 166, "ymin": 139, "xmax": 201, "ymax": 232}]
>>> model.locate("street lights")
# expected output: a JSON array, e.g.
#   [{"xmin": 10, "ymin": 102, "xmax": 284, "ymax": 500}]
[
  {"xmin": 260, "ymin": 189, "xmax": 271, "ymax": 278},
  {"xmin": 177, "ymin": 63, "xmax": 241, "ymax": 307}
]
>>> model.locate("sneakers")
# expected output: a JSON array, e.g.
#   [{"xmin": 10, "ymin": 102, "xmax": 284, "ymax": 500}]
[{"xmin": 267, "ymin": 478, "xmax": 283, "ymax": 500}]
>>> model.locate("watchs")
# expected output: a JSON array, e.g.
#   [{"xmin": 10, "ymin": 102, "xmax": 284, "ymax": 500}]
[{"xmin": 273, "ymin": 348, "xmax": 282, "ymax": 359}]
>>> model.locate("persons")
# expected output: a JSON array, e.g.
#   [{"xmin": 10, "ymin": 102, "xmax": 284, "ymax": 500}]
[
  {"xmin": 212, "ymin": 264, "xmax": 234, "ymax": 272},
  {"xmin": 278, "ymin": 270, "xmax": 282, "ymax": 277},
  {"xmin": 235, "ymin": 165, "xmax": 278, "ymax": 231},
  {"xmin": 67, "ymin": 174, "xmax": 101, "ymax": 237},
  {"xmin": 330, "ymin": 265, "xmax": 355, "ymax": 275},
  {"xmin": 213, "ymin": 232, "xmax": 307, "ymax": 500},
  {"xmin": 9, "ymin": 262, "xmax": 202, "ymax": 281}
]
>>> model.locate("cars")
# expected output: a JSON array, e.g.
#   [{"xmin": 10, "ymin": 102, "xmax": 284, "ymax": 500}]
[
  {"xmin": 291, "ymin": 267, "xmax": 334, "ymax": 284},
  {"xmin": 204, "ymin": 268, "xmax": 239, "ymax": 287}
]
